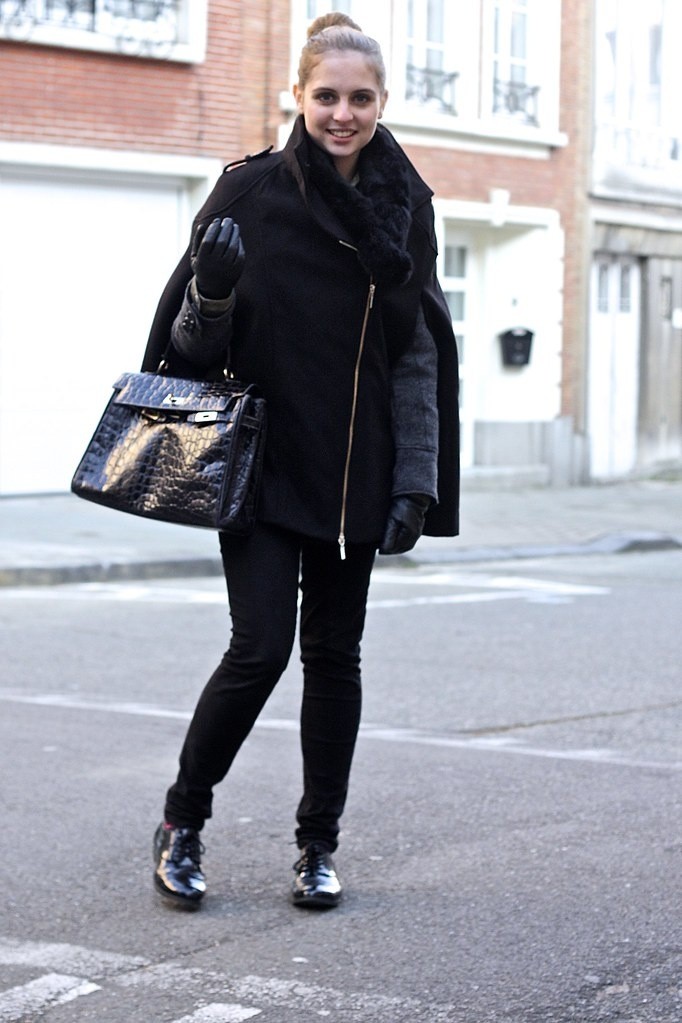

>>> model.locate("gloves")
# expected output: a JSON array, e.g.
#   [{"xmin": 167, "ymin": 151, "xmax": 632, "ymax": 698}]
[
  {"xmin": 190, "ymin": 217, "xmax": 245, "ymax": 300},
  {"xmin": 379, "ymin": 495, "xmax": 430, "ymax": 555}
]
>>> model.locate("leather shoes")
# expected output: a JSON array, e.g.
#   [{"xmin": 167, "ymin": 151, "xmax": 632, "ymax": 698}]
[
  {"xmin": 154, "ymin": 823, "xmax": 206, "ymax": 902},
  {"xmin": 290, "ymin": 843, "xmax": 341, "ymax": 905}
]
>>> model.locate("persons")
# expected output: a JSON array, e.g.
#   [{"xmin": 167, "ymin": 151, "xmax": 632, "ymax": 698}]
[{"xmin": 141, "ymin": 13, "xmax": 458, "ymax": 912}]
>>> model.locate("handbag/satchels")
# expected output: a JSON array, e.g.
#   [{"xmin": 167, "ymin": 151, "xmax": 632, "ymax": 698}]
[{"xmin": 70, "ymin": 323, "xmax": 265, "ymax": 530}]
